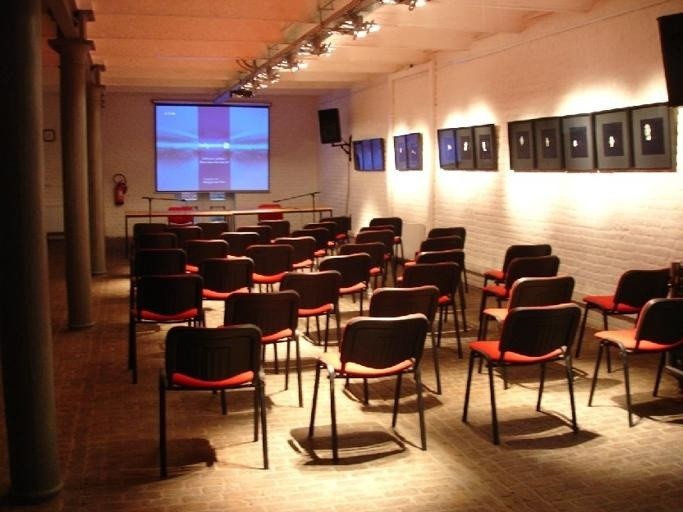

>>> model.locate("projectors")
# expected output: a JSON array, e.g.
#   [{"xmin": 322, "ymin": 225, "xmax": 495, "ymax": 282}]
[{"xmin": 230, "ymin": 90, "xmax": 252, "ymax": 99}]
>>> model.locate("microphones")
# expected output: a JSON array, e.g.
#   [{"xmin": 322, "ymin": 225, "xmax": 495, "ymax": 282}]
[
  {"xmin": 272, "ymin": 199, "xmax": 280, "ymax": 202},
  {"xmin": 177, "ymin": 199, "xmax": 186, "ymax": 202}
]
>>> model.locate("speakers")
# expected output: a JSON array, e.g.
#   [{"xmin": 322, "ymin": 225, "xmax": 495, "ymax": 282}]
[
  {"xmin": 656, "ymin": 12, "xmax": 683, "ymax": 107},
  {"xmin": 318, "ymin": 108, "xmax": 341, "ymax": 144}
]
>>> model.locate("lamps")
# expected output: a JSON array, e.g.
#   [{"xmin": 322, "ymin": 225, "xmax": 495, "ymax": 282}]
[{"xmin": 228, "ymin": 0, "xmax": 430, "ymax": 100}]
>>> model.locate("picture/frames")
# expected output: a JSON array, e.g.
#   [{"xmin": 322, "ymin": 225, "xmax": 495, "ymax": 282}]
[
  {"xmin": 472, "ymin": 123, "xmax": 499, "ymax": 171},
  {"xmin": 454, "ymin": 125, "xmax": 476, "ymax": 170},
  {"xmin": 531, "ymin": 116, "xmax": 566, "ymax": 172},
  {"xmin": 437, "ymin": 128, "xmax": 457, "ymax": 170},
  {"xmin": 353, "ymin": 132, "xmax": 422, "ymax": 170},
  {"xmin": 590, "ymin": 107, "xmax": 632, "ymax": 171},
  {"xmin": 630, "ymin": 101, "xmax": 674, "ymax": 170},
  {"xmin": 43, "ymin": 128, "xmax": 55, "ymax": 142},
  {"xmin": 560, "ymin": 112, "xmax": 597, "ymax": 173},
  {"xmin": 507, "ymin": 119, "xmax": 540, "ymax": 172}
]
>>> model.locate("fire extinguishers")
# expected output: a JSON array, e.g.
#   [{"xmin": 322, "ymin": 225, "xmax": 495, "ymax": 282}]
[{"xmin": 113, "ymin": 173, "xmax": 127, "ymax": 205}]
